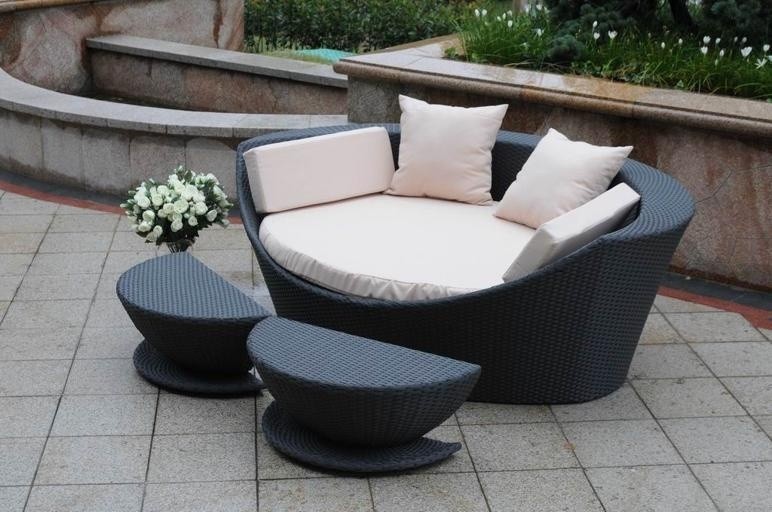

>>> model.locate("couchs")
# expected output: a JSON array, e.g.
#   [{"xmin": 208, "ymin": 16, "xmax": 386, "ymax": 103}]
[{"xmin": 233, "ymin": 121, "xmax": 698, "ymax": 408}]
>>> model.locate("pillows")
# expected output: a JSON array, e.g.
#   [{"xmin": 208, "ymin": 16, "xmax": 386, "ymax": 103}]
[
  {"xmin": 492, "ymin": 126, "xmax": 634, "ymax": 231},
  {"xmin": 382, "ymin": 93, "xmax": 511, "ymax": 206}
]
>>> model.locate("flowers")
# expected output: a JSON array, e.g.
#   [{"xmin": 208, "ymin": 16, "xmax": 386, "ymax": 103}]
[{"xmin": 118, "ymin": 162, "xmax": 232, "ymax": 251}]
